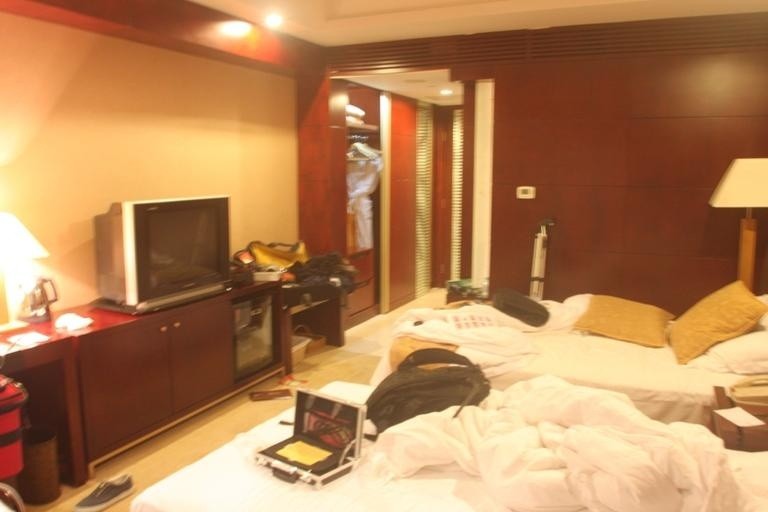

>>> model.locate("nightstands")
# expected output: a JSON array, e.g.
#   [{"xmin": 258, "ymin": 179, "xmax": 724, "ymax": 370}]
[{"xmin": 698, "ymin": 384, "xmax": 768, "ymax": 453}]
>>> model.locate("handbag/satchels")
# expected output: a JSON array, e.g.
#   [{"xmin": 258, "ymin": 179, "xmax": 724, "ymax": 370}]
[{"xmin": 247, "ymin": 240, "xmax": 309, "ymax": 269}]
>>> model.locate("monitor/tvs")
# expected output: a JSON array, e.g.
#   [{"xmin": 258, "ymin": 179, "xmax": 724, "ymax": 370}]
[{"xmin": 94, "ymin": 195, "xmax": 231, "ymax": 316}]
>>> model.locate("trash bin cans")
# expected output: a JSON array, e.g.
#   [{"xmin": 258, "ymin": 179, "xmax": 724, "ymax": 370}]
[
  {"xmin": 447, "ymin": 278, "xmax": 490, "ymax": 303},
  {"xmin": 18, "ymin": 428, "xmax": 61, "ymax": 505}
]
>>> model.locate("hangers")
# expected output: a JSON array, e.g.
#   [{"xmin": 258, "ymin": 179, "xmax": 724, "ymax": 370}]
[{"xmin": 344, "ymin": 134, "xmax": 383, "ymax": 162}]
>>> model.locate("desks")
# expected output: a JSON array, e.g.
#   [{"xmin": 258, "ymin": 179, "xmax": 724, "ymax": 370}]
[
  {"xmin": 285, "ymin": 278, "xmax": 347, "ymax": 348},
  {"xmin": 0, "ymin": 318, "xmax": 88, "ymax": 488}
]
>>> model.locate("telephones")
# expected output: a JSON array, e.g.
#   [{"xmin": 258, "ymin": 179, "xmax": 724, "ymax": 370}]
[{"xmin": 730, "ymin": 374, "xmax": 768, "ymax": 415}]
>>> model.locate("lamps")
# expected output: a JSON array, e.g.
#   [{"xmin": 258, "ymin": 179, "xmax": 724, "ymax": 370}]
[{"xmin": 709, "ymin": 158, "xmax": 768, "ymax": 295}]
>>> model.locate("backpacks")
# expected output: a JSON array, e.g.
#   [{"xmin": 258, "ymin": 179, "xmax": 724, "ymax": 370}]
[
  {"xmin": 492, "ymin": 287, "xmax": 552, "ymax": 327},
  {"xmin": 363, "ymin": 348, "xmax": 491, "ymax": 442}
]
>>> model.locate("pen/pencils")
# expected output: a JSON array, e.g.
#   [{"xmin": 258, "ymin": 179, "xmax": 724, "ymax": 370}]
[{"xmin": 279, "ymin": 421, "xmax": 294, "ymax": 426}]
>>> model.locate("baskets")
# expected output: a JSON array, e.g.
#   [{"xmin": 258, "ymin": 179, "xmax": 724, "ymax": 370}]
[{"xmin": 291, "ymin": 324, "xmax": 327, "ymax": 363}]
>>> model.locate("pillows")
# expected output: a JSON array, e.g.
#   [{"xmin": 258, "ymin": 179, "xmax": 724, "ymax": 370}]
[{"xmin": 571, "ymin": 278, "xmax": 766, "ymax": 365}]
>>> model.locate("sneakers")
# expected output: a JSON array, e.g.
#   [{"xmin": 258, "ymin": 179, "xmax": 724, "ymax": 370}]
[{"xmin": 72, "ymin": 472, "xmax": 135, "ymax": 512}]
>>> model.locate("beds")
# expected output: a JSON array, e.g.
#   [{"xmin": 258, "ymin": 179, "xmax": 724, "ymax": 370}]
[
  {"xmin": 375, "ymin": 296, "xmax": 767, "ymax": 426},
  {"xmin": 132, "ymin": 379, "xmax": 768, "ymax": 512}
]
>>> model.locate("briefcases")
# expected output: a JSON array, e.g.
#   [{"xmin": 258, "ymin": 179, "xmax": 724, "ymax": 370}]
[{"xmin": 254, "ymin": 388, "xmax": 368, "ymax": 489}]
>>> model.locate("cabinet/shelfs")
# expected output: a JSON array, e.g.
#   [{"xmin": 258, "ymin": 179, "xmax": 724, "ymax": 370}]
[
  {"xmin": 296, "ymin": 79, "xmax": 416, "ymax": 332},
  {"xmin": 30, "ymin": 272, "xmax": 285, "ymax": 479}
]
